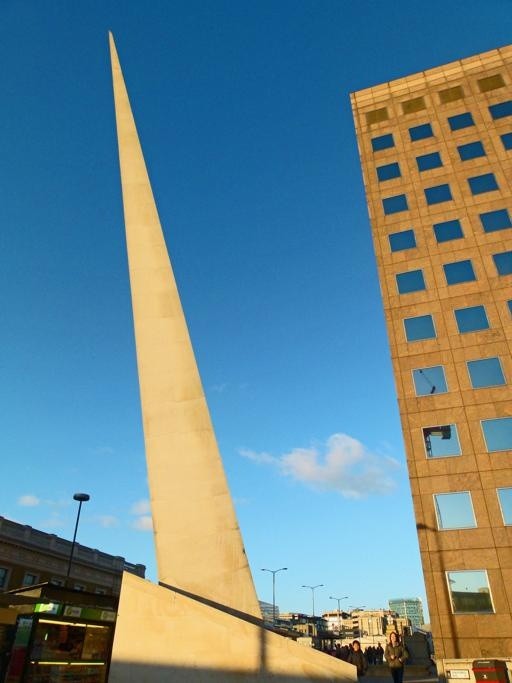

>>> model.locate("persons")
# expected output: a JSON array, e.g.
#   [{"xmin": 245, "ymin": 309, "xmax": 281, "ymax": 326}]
[
  {"xmin": 348, "ymin": 640, "xmax": 368, "ymax": 677},
  {"xmin": 323, "ymin": 641, "xmax": 384, "ymax": 665},
  {"xmin": 385, "ymin": 631, "xmax": 409, "ymax": 682}
]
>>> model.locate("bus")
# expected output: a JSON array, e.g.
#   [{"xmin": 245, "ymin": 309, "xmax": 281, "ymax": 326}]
[{"xmin": 270, "ymin": 617, "xmax": 294, "ymax": 630}]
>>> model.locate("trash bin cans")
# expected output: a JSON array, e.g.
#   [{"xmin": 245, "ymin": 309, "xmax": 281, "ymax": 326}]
[{"xmin": 472, "ymin": 660, "xmax": 509, "ymax": 683}]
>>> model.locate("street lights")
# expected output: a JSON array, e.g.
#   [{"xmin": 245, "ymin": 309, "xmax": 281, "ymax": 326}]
[
  {"xmin": 53, "ymin": 491, "xmax": 91, "ymax": 587},
  {"xmin": 260, "ymin": 567, "xmax": 288, "ymax": 626},
  {"xmin": 347, "ymin": 604, "xmax": 367, "ymax": 647},
  {"xmin": 328, "ymin": 595, "xmax": 349, "ymax": 647},
  {"xmin": 301, "ymin": 584, "xmax": 324, "ymax": 638}
]
